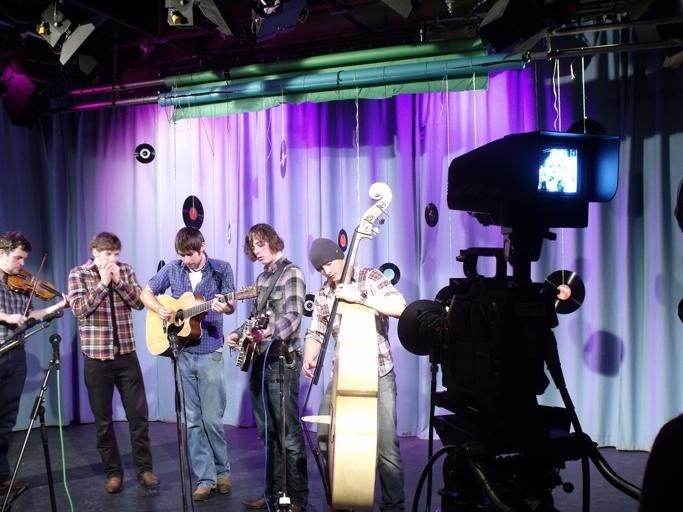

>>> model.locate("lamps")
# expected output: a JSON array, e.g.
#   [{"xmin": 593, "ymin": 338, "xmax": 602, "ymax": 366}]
[
  {"xmin": 447, "ymin": 1, "xmax": 512, "ymax": 39},
  {"xmin": 165, "ymin": 0, "xmax": 236, "ymax": 39},
  {"xmin": 36, "ymin": 2, "xmax": 105, "ymax": 66},
  {"xmin": 372, "ymin": 2, "xmax": 417, "ymax": 31},
  {"xmin": 251, "ymin": 1, "xmax": 309, "ymax": 44}
]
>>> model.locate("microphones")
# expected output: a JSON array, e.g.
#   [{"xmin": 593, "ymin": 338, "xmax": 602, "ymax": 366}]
[
  {"xmin": 46, "ymin": 334, "xmax": 63, "ymax": 371},
  {"xmin": 282, "ymin": 341, "xmax": 295, "ymax": 370},
  {"xmin": 166, "ymin": 328, "xmax": 183, "ymax": 355}
]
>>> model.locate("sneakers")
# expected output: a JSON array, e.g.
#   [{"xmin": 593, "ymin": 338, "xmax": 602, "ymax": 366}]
[
  {"xmin": 217, "ymin": 476, "xmax": 231, "ymax": 495},
  {"xmin": 192, "ymin": 487, "xmax": 216, "ymax": 501}
]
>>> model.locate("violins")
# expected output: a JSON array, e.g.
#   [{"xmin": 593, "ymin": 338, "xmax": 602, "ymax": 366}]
[{"xmin": 8, "ymin": 268, "xmax": 62, "ymax": 301}]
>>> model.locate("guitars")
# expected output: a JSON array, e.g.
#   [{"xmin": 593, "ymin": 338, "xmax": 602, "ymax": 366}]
[
  {"xmin": 145, "ymin": 286, "xmax": 257, "ymax": 356},
  {"xmin": 235, "ymin": 314, "xmax": 269, "ymax": 371}
]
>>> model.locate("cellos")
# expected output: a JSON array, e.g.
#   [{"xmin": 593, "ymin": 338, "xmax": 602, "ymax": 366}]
[{"xmin": 301, "ymin": 182, "xmax": 393, "ymax": 512}]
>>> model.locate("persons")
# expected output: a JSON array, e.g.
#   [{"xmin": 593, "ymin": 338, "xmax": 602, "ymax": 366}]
[
  {"xmin": 301, "ymin": 238, "xmax": 407, "ymax": 512},
  {"xmin": 1, "ymin": 232, "xmax": 67, "ymax": 497},
  {"xmin": 140, "ymin": 227, "xmax": 235, "ymax": 500},
  {"xmin": 68, "ymin": 233, "xmax": 159, "ymax": 493},
  {"xmin": 227, "ymin": 223, "xmax": 307, "ymax": 512}
]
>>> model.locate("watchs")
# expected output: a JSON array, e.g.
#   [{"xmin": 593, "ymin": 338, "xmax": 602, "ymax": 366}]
[{"xmin": 359, "ymin": 292, "xmax": 367, "ymax": 306}]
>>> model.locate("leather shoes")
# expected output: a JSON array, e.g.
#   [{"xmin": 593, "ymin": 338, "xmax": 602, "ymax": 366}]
[
  {"xmin": 138, "ymin": 470, "xmax": 160, "ymax": 487},
  {"xmin": 106, "ymin": 476, "xmax": 123, "ymax": 493},
  {"xmin": 242, "ymin": 497, "xmax": 268, "ymax": 509}
]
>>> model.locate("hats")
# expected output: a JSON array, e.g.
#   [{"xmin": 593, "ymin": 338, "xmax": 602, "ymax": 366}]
[{"xmin": 310, "ymin": 239, "xmax": 344, "ymax": 271}]
